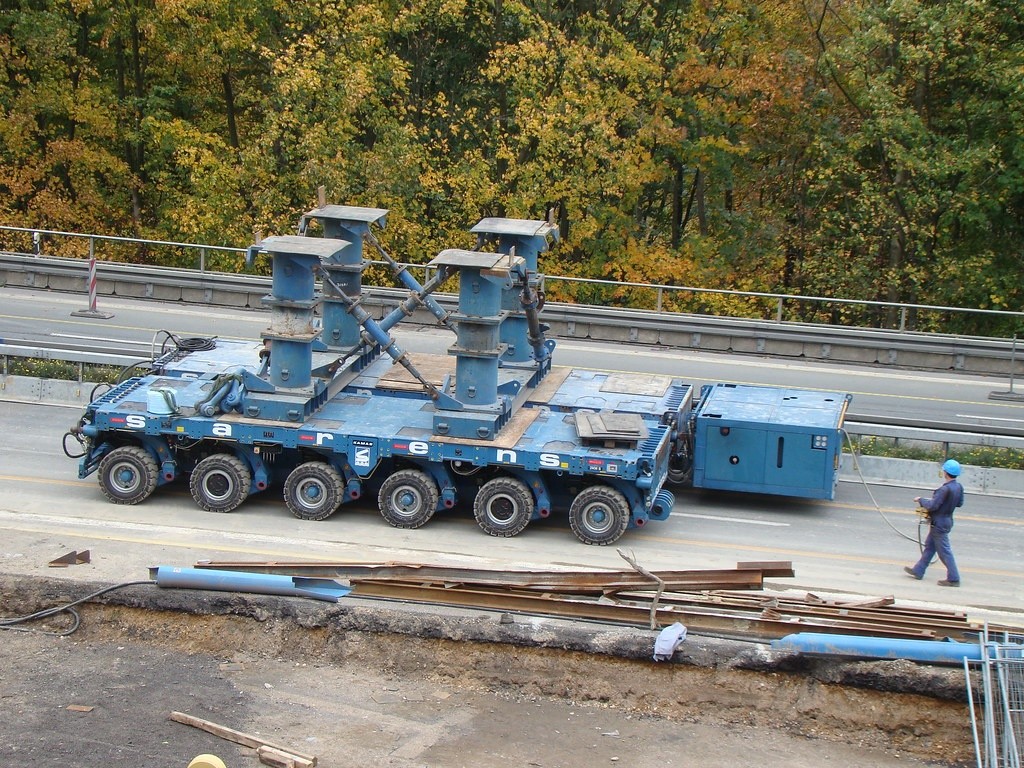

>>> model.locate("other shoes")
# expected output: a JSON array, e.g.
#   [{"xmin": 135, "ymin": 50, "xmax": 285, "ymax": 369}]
[
  {"xmin": 938, "ymin": 579, "xmax": 960, "ymax": 588},
  {"xmin": 904, "ymin": 566, "xmax": 925, "ymax": 579}
]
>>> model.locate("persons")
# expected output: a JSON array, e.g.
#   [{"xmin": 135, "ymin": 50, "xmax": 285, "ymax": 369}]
[{"xmin": 904, "ymin": 459, "xmax": 965, "ymax": 587}]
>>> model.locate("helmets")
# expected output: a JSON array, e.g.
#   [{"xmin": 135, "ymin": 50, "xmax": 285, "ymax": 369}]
[{"xmin": 942, "ymin": 460, "xmax": 962, "ymax": 476}]
